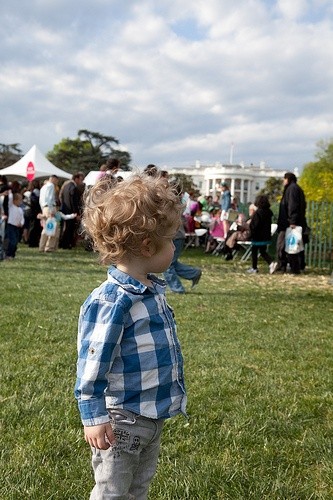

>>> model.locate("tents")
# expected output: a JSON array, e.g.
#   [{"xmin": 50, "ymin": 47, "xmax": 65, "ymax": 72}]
[{"xmin": 0, "ymin": 145, "xmax": 133, "ymax": 190}]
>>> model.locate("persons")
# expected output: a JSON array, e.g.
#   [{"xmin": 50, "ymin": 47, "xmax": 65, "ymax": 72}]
[
  {"xmin": 0, "ymin": 164, "xmax": 307, "ymax": 293},
  {"xmin": 73, "ymin": 172, "xmax": 188, "ymax": 500}
]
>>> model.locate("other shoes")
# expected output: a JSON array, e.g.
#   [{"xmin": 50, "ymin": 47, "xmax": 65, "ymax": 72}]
[
  {"xmin": 246, "ymin": 267, "xmax": 258, "ymax": 273},
  {"xmin": 204, "ymin": 248, "xmax": 233, "ymax": 260},
  {"xmin": 189, "ymin": 269, "xmax": 202, "ymax": 291},
  {"xmin": 165, "ymin": 289, "xmax": 187, "ymax": 295},
  {"xmin": 268, "ymin": 262, "xmax": 279, "ymax": 275}
]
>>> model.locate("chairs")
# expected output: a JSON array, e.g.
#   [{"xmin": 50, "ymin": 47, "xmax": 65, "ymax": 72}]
[{"xmin": 184, "ymin": 220, "xmax": 278, "ymax": 264}]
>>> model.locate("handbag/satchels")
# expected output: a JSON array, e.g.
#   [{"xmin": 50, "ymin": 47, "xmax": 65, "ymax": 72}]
[
  {"xmin": 41, "ymin": 217, "xmax": 57, "ymax": 237},
  {"xmin": 284, "ymin": 226, "xmax": 304, "ymax": 254}
]
